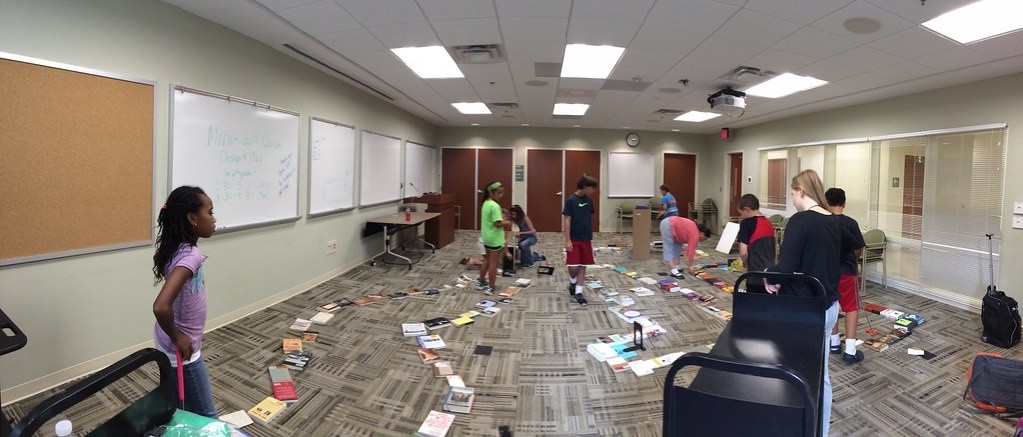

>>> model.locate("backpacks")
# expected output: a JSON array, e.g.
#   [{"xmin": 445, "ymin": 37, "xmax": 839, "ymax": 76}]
[{"xmin": 964, "ymin": 352, "xmax": 1023, "ymax": 419}]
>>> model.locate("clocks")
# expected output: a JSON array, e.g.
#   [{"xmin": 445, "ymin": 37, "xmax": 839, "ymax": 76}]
[{"xmin": 625, "ymin": 133, "xmax": 640, "ymax": 147}]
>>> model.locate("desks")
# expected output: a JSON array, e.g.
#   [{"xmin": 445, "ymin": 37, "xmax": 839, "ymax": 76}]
[{"xmin": 364, "ymin": 209, "xmax": 442, "ymax": 269}]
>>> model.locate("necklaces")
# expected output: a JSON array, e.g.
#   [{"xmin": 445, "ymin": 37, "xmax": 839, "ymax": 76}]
[{"xmin": 808, "ymin": 205, "xmax": 818, "ymax": 210}]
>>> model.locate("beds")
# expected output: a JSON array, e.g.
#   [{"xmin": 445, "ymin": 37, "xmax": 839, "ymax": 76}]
[{"xmin": 662, "ymin": 270, "xmax": 829, "ymax": 437}]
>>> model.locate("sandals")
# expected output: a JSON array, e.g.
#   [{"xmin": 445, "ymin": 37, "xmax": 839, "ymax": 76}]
[
  {"xmin": 843, "ymin": 350, "xmax": 864, "ymax": 362},
  {"xmin": 831, "ymin": 343, "xmax": 842, "ymax": 354}
]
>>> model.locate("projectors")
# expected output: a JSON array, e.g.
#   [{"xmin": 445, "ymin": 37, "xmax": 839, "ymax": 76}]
[{"xmin": 711, "ymin": 97, "xmax": 746, "ymax": 114}]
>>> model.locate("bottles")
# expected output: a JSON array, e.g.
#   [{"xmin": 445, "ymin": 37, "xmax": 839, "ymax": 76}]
[
  {"xmin": 54, "ymin": 420, "xmax": 75, "ymax": 437},
  {"xmin": 405, "ymin": 206, "xmax": 411, "ymax": 221}
]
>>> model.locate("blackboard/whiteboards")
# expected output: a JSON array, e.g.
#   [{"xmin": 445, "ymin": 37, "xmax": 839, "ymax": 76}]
[
  {"xmin": 715, "ymin": 222, "xmax": 740, "ymax": 254},
  {"xmin": 359, "ymin": 129, "xmax": 403, "ymax": 209},
  {"xmin": 306, "ymin": 116, "xmax": 357, "ymax": 219},
  {"xmin": 167, "ymin": 84, "xmax": 303, "ymax": 237},
  {"xmin": 404, "ymin": 140, "xmax": 437, "ymax": 198},
  {"xmin": 608, "ymin": 151, "xmax": 655, "ymax": 198}
]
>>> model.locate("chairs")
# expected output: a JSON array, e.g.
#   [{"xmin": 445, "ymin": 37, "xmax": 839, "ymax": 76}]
[
  {"xmin": 767, "ymin": 213, "xmax": 791, "ymax": 264},
  {"xmin": 857, "ymin": 227, "xmax": 889, "ymax": 292},
  {"xmin": 617, "ymin": 197, "xmax": 716, "ymax": 236}
]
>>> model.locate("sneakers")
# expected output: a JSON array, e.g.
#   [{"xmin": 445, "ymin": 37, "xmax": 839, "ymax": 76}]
[
  {"xmin": 477, "ymin": 281, "xmax": 490, "ymax": 289},
  {"xmin": 485, "ymin": 286, "xmax": 496, "ymax": 295},
  {"xmin": 570, "ymin": 282, "xmax": 578, "ymax": 296},
  {"xmin": 574, "ymin": 293, "xmax": 588, "ymax": 306}
]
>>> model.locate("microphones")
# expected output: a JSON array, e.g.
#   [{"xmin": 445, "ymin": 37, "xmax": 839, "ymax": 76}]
[{"xmin": 410, "ymin": 183, "xmax": 421, "ymax": 194}]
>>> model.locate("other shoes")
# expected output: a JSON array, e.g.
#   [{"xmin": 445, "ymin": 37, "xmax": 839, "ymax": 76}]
[
  {"xmin": 671, "ymin": 273, "xmax": 684, "ymax": 279},
  {"xmin": 533, "ymin": 251, "xmax": 546, "ymax": 261},
  {"xmin": 522, "ymin": 263, "xmax": 534, "ymax": 268},
  {"xmin": 679, "ymin": 269, "xmax": 683, "ymax": 272}
]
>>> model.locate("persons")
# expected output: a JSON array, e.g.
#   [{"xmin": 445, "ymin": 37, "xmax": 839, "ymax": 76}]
[
  {"xmin": 659, "ymin": 216, "xmax": 712, "ymax": 279},
  {"xmin": 657, "ymin": 185, "xmax": 678, "ymax": 220},
  {"xmin": 764, "ymin": 169, "xmax": 857, "ymax": 437},
  {"xmin": 562, "ymin": 173, "xmax": 599, "ymax": 306},
  {"xmin": 476, "ymin": 182, "xmax": 546, "ymax": 296},
  {"xmin": 153, "ymin": 185, "xmax": 219, "ymax": 419},
  {"xmin": 737, "ymin": 194, "xmax": 776, "ymax": 293},
  {"xmin": 825, "ymin": 188, "xmax": 864, "ymax": 363}
]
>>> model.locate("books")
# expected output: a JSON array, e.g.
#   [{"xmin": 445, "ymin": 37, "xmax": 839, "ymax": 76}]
[
  {"xmin": 268, "ymin": 365, "xmax": 298, "ymax": 403},
  {"xmin": 401, "ymin": 315, "xmax": 476, "ymax": 437},
  {"xmin": 451, "ymin": 271, "xmax": 533, "ymax": 329},
  {"xmin": 282, "ymin": 318, "xmax": 319, "ymax": 371},
  {"xmin": 583, "ymin": 272, "xmax": 716, "ymax": 377},
  {"xmin": 248, "ymin": 396, "xmax": 287, "ymax": 426},
  {"xmin": 310, "ymin": 295, "xmax": 379, "ymax": 325},
  {"xmin": 838, "ymin": 298, "xmax": 925, "ymax": 352},
  {"xmin": 368, "ymin": 283, "xmax": 466, "ymax": 299},
  {"xmin": 606, "ymin": 240, "xmax": 746, "ymax": 321}
]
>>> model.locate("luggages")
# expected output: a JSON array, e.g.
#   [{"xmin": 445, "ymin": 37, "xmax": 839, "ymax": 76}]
[{"xmin": 981, "ymin": 234, "xmax": 1022, "ymax": 349}]
